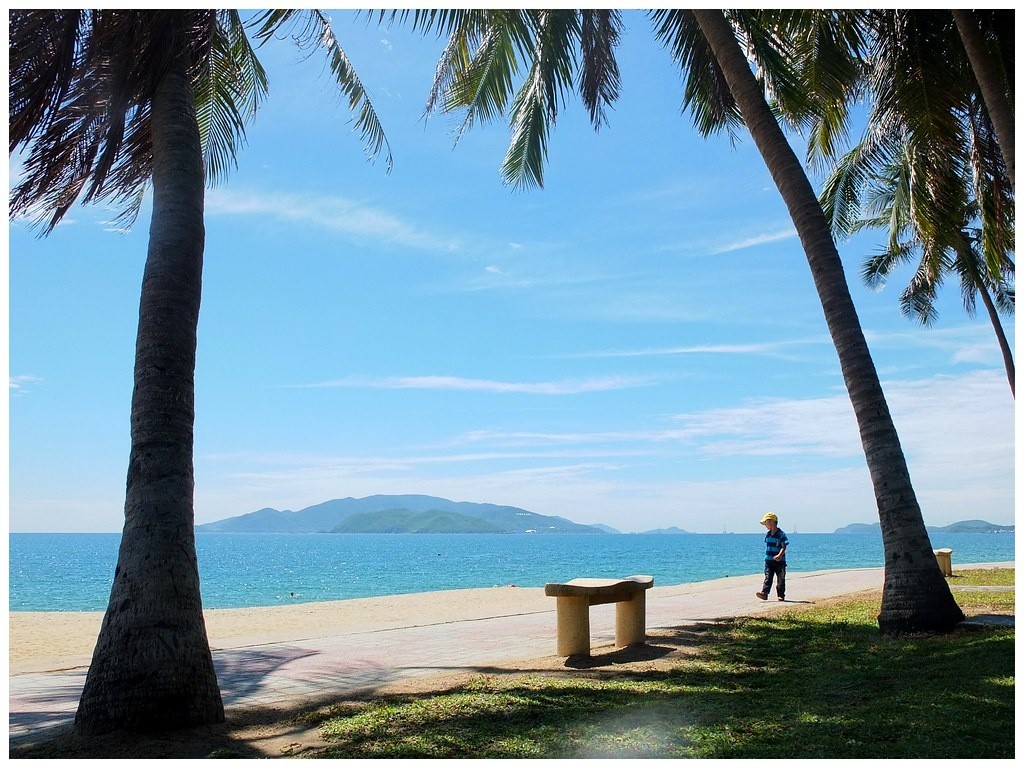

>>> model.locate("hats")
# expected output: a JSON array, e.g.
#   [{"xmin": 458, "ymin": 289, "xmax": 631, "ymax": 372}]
[{"xmin": 760, "ymin": 512, "xmax": 778, "ymax": 525}]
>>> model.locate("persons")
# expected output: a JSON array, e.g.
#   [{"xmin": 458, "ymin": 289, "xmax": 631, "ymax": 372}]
[{"xmin": 756, "ymin": 512, "xmax": 789, "ymax": 601}]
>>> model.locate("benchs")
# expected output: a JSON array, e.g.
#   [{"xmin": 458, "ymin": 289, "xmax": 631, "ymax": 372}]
[
  {"xmin": 545, "ymin": 575, "xmax": 654, "ymax": 657},
  {"xmin": 933, "ymin": 548, "xmax": 954, "ymax": 577}
]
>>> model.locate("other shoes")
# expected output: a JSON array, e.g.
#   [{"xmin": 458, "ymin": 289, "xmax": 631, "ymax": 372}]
[
  {"xmin": 778, "ymin": 597, "xmax": 784, "ymax": 601},
  {"xmin": 756, "ymin": 591, "xmax": 768, "ymax": 600}
]
